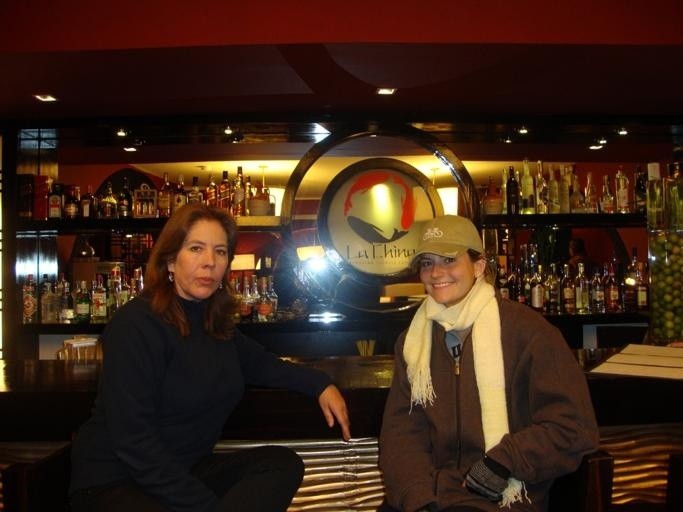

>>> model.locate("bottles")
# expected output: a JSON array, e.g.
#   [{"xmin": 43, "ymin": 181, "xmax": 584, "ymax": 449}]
[
  {"xmin": 482, "ymin": 159, "xmax": 630, "ymax": 214},
  {"xmin": 22, "ymin": 264, "xmax": 142, "ymax": 324},
  {"xmin": 157, "ymin": 167, "xmax": 270, "ymax": 218},
  {"xmin": 228, "ymin": 271, "xmax": 279, "ymax": 320},
  {"xmin": 48, "ymin": 177, "xmax": 132, "ymax": 219},
  {"xmin": 497, "ymin": 238, "xmax": 648, "ymax": 316}
]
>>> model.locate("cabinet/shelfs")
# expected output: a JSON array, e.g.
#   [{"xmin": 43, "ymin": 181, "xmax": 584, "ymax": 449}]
[{"xmin": 1, "ymin": 110, "xmax": 683, "ymax": 358}]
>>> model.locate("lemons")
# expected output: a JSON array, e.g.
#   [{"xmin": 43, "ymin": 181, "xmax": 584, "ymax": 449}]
[{"xmin": 649, "ymin": 234, "xmax": 683, "ymax": 347}]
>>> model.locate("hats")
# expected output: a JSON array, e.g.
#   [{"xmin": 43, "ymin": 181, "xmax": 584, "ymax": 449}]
[{"xmin": 412, "ymin": 215, "xmax": 482, "ymax": 262}]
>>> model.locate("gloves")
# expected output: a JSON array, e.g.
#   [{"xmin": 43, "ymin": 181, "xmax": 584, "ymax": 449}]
[{"xmin": 463, "ymin": 453, "xmax": 512, "ymax": 503}]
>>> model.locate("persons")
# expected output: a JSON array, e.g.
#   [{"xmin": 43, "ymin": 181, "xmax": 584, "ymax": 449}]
[
  {"xmin": 376, "ymin": 215, "xmax": 600, "ymax": 510},
  {"xmin": 60, "ymin": 201, "xmax": 351, "ymax": 512}
]
